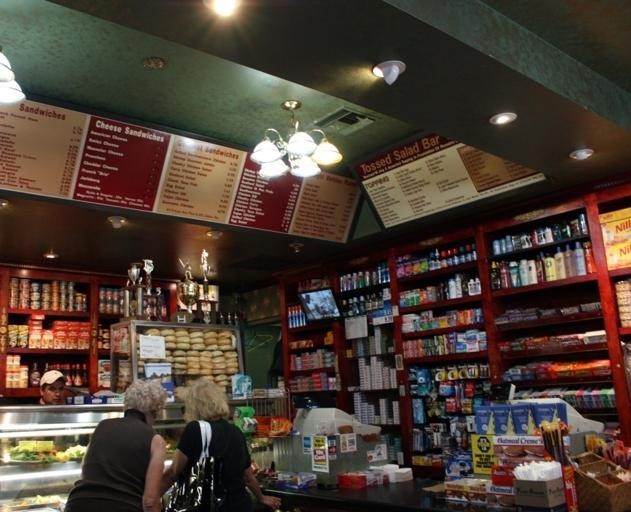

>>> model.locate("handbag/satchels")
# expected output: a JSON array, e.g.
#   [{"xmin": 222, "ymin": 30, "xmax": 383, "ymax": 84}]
[{"xmin": 165, "ymin": 420, "xmax": 228, "ymax": 512}]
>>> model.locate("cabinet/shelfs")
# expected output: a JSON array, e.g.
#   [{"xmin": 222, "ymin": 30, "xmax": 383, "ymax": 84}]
[
  {"xmin": 271, "ymin": 181, "xmax": 631, "ymax": 479},
  {"xmin": 0, "ymin": 262, "xmax": 246, "ymax": 398},
  {"xmin": 1, "ymin": 403, "xmax": 187, "ymax": 512}
]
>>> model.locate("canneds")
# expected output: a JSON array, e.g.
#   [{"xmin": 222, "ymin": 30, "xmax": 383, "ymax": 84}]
[
  {"xmin": 98, "ymin": 287, "xmax": 167, "ymax": 316},
  {"xmin": 9, "ymin": 277, "xmax": 88, "ymax": 312},
  {"xmin": 614, "ymin": 279, "xmax": 631, "ymax": 327}
]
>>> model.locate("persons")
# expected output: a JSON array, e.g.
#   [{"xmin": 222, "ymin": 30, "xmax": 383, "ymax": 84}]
[
  {"xmin": 64, "ymin": 379, "xmax": 167, "ymax": 512},
  {"xmin": 39, "ymin": 370, "xmax": 66, "ymax": 405},
  {"xmin": 161, "ymin": 379, "xmax": 282, "ymax": 512}
]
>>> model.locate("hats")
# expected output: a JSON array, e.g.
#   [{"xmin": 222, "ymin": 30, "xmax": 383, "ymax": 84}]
[{"xmin": 40, "ymin": 370, "xmax": 68, "ymax": 386}]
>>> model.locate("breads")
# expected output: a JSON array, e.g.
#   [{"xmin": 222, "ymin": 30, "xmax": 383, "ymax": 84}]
[{"xmin": 116, "ymin": 328, "xmax": 240, "ymax": 392}]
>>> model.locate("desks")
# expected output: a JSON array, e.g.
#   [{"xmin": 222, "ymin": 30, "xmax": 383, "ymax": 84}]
[{"xmin": 244, "ymin": 473, "xmax": 606, "ymax": 511}]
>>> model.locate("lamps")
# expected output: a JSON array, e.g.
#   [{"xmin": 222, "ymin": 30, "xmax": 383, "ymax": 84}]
[
  {"xmin": 372, "ymin": 60, "xmax": 406, "ymax": 85},
  {"xmin": 0, "ymin": 44, "xmax": 26, "ymax": 104},
  {"xmin": 250, "ymin": 101, "xmax": 343, "ymax": 179}
]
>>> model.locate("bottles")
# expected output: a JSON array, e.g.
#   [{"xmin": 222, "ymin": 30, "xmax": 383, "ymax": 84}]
[
  {"xmin": 30, "ymin": 362, "xmax": 87, "ymax": 388},
  {"xmin": 100, "ymin": 287, "xmax": 167, "ymax": 318},
  {"xmin": 340, "ymin": 214, "xmax": 595, "ymax": 318}
]
